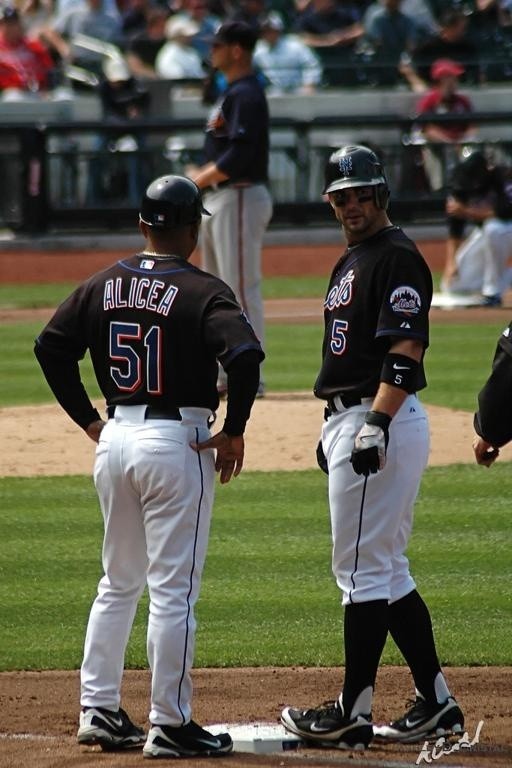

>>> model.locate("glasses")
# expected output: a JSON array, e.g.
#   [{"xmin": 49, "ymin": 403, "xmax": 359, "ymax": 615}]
[{"xmin": 328, "ymin": 186, "xmax": 373, "ymax": 207}]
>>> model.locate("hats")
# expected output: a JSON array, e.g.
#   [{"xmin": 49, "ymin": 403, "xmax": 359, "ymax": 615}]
[
  {"xmin": 432, "ymin": 60, "xmax": 467, "ymax": 80},
  {"xmin": 198, "ymin": 21, "xmax": 257, "ymax": 50}
]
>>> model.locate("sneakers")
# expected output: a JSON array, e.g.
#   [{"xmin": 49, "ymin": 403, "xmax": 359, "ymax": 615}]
[
  {"xmin": 280, "ymin": 700, "xmax": 374, "ymax": 751},
  {"xmin": 142, "ymin": 719, "xmax": 233, "ymax": 759},
  {"xmin": 76, "ymin": 706, "xmax": 146, "ymax": 751},
  {"xmin": 373, "ymin": 696, "xmax": 466, "ymax": 744}
]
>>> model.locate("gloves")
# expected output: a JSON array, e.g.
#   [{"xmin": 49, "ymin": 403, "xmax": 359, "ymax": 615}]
[
  {"xmin": 316, "ymin": 436, "xmax": 329, "ymax": 476},
  {"xmin": 349, "ymin": 411, "xmax": 392, "ymax": 478}
]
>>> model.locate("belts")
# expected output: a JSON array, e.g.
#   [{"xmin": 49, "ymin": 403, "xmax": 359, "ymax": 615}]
[
  {"xmin": 106, "ymin": 405, "xmax": 211, "ymax": 429},
  {"xmin": 324, "ymin": 385, "xmax": 416, "ymax": 421}
]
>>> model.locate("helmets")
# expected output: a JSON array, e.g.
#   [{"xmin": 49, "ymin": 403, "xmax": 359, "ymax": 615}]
[
  {"xmin": 321, "ymin": 144, "xmax": 391, "ymax": 211},
  {"xmin": 139, "ymin": 174, "xmax": 211, "ymax": 229}
]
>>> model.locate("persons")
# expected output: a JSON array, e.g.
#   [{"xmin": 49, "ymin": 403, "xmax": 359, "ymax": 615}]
[
  {"xmin": 2, "ymin": 0, "xmax": 512, "ymax": 203},
  {"xmin": 439, "ymin": 148, "xmax": 512, "ymax": 307},
  {"xmin": 474, "ymin": 320, "xmax": 512, "ymax": 466},
  {"xmin": 282, "ymin": 143, "xmax": 465, "ymax": 750},
  {"xmin": 184, "ymin": 19, "xmax": 274, "ymax": 397},
  {"xmin": 33, "ymin": 175, "xmax": 265, "ymax": 757}
]
